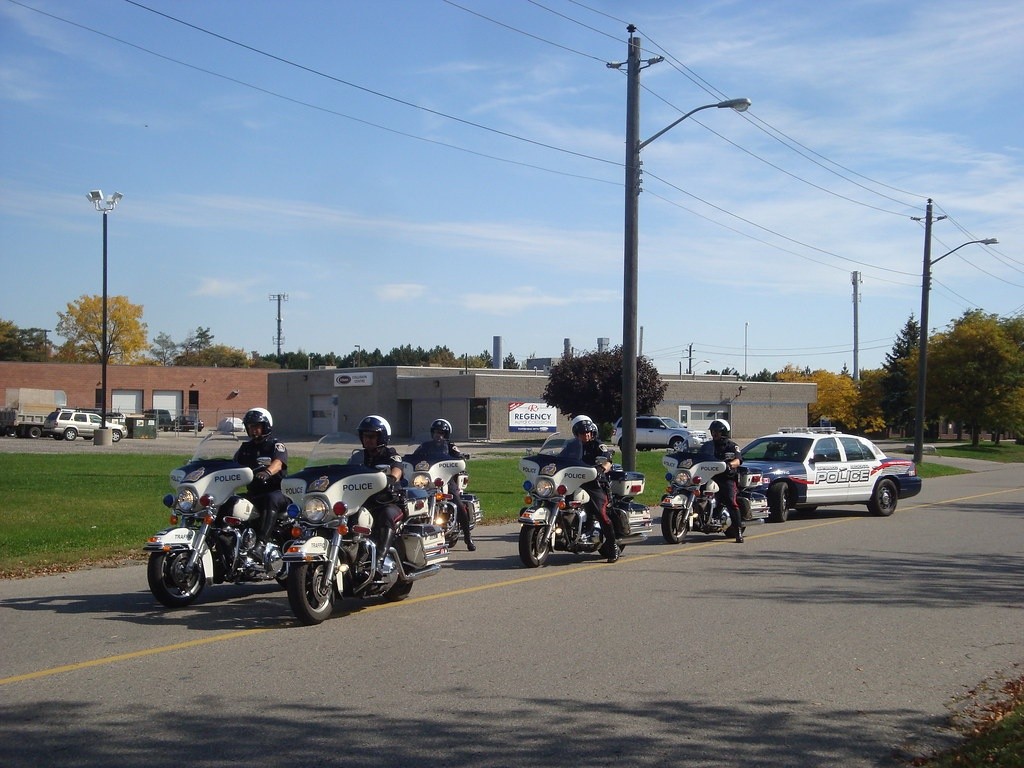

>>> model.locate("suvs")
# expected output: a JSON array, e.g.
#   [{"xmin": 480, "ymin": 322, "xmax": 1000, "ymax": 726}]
[
  {"xmin": 143, "ymin": 409, "xmax": 171, "ymax": 433},
  {"xmin": 43, "ymin": 409, "xmax": 129, "ymax": 443},
  {"xmin": 610, "ymin": 414, "xmax": 710, "ymax": 453}
]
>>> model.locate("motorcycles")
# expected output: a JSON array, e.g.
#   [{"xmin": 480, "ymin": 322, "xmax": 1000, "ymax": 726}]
[
  {"xmin": 260, "ymin": 431, "xmax": 451, "ymax": 625},
  {"xmin": 656, "ymin": 435, "xmax": 773, "ymax": 545},
  {"xmin": 396, "ymin": 436, "xmax": 482, "ymax": 550},
  {"xmin": 141, "ymin": 430, "xmax": 299, "ymax": 609},
  {"xmin": 516, "ymin": 433, "xmax": 655, "ymax": 568}
]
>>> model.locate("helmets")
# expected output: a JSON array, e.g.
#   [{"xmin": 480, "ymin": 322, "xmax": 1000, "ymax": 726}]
[
  {"xmin": 356, "ymin": 414, "xmax": 392, "ymax": 448},
  {"xmin": 592, "ymin": 423, "xmax": 599, "ymax": 439},
  {"xmin": 430, "ymin": 418, "xmax": 453, "ymax": 440},
  {"xmin": 242, "ymin": 407, "xmax": 274, "ymax": 435},
  {"xmin": 707, "ymin": 419, "xmax": 731, "ymax": 432},
  {"xmin": 570, "ymin": 415, "xmax": 594, "ymax": 439}
]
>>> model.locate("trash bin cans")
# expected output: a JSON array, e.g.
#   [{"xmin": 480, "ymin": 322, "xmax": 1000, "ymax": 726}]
[{"xmin": 125, "ymin": 417, "xmax": 156, "ymax": 439}]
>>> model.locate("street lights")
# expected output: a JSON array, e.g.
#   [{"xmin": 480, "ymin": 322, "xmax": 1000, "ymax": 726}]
[
  {"xmin": 619, "ymin": 97, "xmax": 750, "ymax": 482},
  {"xmin": 744, "ymin": 322, "xmax": 750, "ymax": 378},
  {"xmin": 687, "ymin": 360, "xmax": 709, "ymax": 375},
  {"xmin": 85, "ymin": 188, "xmax": 124, "ymax": 448},
  {"xmin": 913, "ymin": 239, "xmax": 1001, "ymax": 468}
]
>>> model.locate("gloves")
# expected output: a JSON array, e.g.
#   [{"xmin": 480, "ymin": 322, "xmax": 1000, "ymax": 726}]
[
  {"xmin": 725, "ymin": 463, "xmax": 732, "ymax": 471},
  {"xmin": 255, "ymin": 469, "xmax": 271, "ymax": 482},
  {"xmin": 384, "ymin": 474, "xmax": 397, "ymax": 492},
  {"xmin": 596, "ymin": 465, "xmax": 606, "ymax": 479}
]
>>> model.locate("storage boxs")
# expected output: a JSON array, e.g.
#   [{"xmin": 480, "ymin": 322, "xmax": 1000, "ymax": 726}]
[
  {"xmin": 610, "ymin": 471, "xmax": 646, "ymax": 496},
  {"xmin": 401, "ymin": 486, "xmax": 429, "ymax": 517},
  {"xmin": 402, "ymin": 524, "xmax": 447, "ymax": 567},
  {"xmin": 461, "ymin": 494, "xmax": 482, "ymax": 523},
  {"xmin": 455, "ymin": 471, "xmax": 469, "ymax": 489},
  {"xmin": 614, "ymin": 503, "xmax": 653, "ymax": 536},
  {"xmin": 739, "ymin": 464, "xmax": 763, "ymax": 487},
  {"xmin": 737, "ymin": 496, "xmax": 769, "ymax": 520}
]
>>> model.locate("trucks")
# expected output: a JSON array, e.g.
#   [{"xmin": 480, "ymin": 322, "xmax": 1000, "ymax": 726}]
[{"xmin": 3, "ymin": 408, "xmax": 75, "ymax": 440}]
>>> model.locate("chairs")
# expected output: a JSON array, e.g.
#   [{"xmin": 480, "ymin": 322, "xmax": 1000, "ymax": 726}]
[{"xmin": 783, "ymin": 445, "xmax": 796, "ymax": 455}]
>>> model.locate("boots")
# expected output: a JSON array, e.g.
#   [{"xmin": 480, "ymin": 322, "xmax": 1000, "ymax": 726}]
[
  {"xmin": 249, "ymin": 508, "xmax": 279, "ymax": 562},
  {"xmin": 601, "ymin": 523, "xmax": 619, "ymax": 563},
  {"xmin": 369, "ymin": 526, "xmax": 394, "ymax": 581},
  {"xmin": 463, "ymin": 512, "xmax": 476, "ymax": 552},
  {"xmin": 729, "ymin": 509, "xmax": 744, "ymax": 543}
]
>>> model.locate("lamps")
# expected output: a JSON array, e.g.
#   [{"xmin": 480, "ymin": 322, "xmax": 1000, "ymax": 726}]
[
  {"xmin": 303, "ymin": 375, "xmax": 307, "ymax": 380},
  {"xmin": 736, "ymin": 385, "xmax": 748, "ymax": 397},
  {"xmin": 435, "ymin": 380, "xmax": 439, "ymax": 387}
]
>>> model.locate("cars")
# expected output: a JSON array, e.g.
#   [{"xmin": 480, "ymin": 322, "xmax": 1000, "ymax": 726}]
[
  {"xmin": 220, "ymin": 417, "xmax": 245, "ymax": 433},
  {"xmin": 722, "ymin": 426, "xmax": 925, "ymax": 523},
  {"xmin": 170, "ymin": 414, "xmax": 204, "ymax": 432}
]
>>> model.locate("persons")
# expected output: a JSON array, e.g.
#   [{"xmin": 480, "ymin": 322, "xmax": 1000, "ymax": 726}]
[
  {"xmin": 347, "ymin": 414, "xmax": 405, "ymax": 580},
  {"xmin": 232, "ymin": 407, "xmax": 288, "ymax": 552},
  {"xmin": 558, "ymin": 415, "xmax": 621, "ymax": 563},
  {"xmin": 412, "ymin": 418, "xmax": 476, "ymax": 551},
  {"xmin": 697, "ymin": 419, "xmax": 744, "ymax": 543}
]
